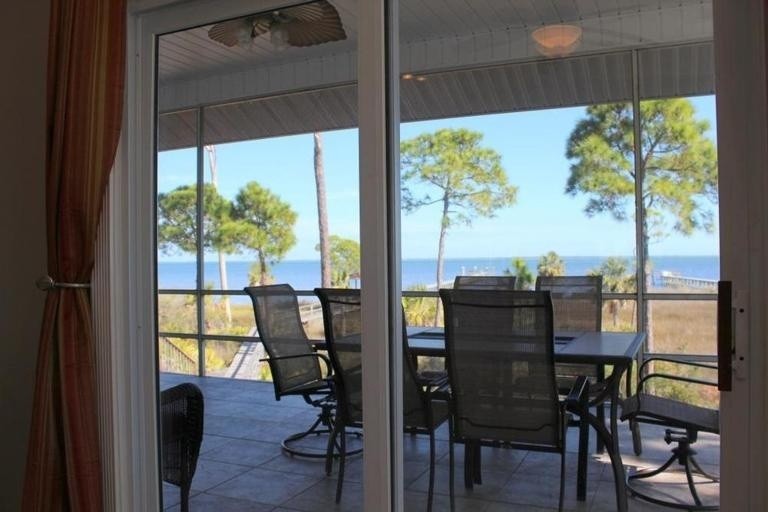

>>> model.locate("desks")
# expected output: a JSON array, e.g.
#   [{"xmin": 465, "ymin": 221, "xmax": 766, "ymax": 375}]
[{"xmin": 314, "ymin": 325, "xmax": 649, "ymax": 512}]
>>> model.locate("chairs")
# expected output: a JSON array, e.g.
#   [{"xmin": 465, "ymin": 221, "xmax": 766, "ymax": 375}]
[
  {"xmin": 242, "ymin": 281, "xmax": 364, "ymax": 461},
  {"xmin": 452, "ymin": 275, "xmax": 518, "ymax": 290},
  {"xmin": 313, "ymin": 285, "xmax": 450, "ymax": 512},
  {"xmin": 621, "ymin": 354, "xmax": 719, "ymax": 508},
  {"xmin": 512, "ymin": 275, "xmax": 606, "ymax": 454},
  {"xmin": 438, "ymin": 287, "xmax": 593, "ymax": 512}
]
